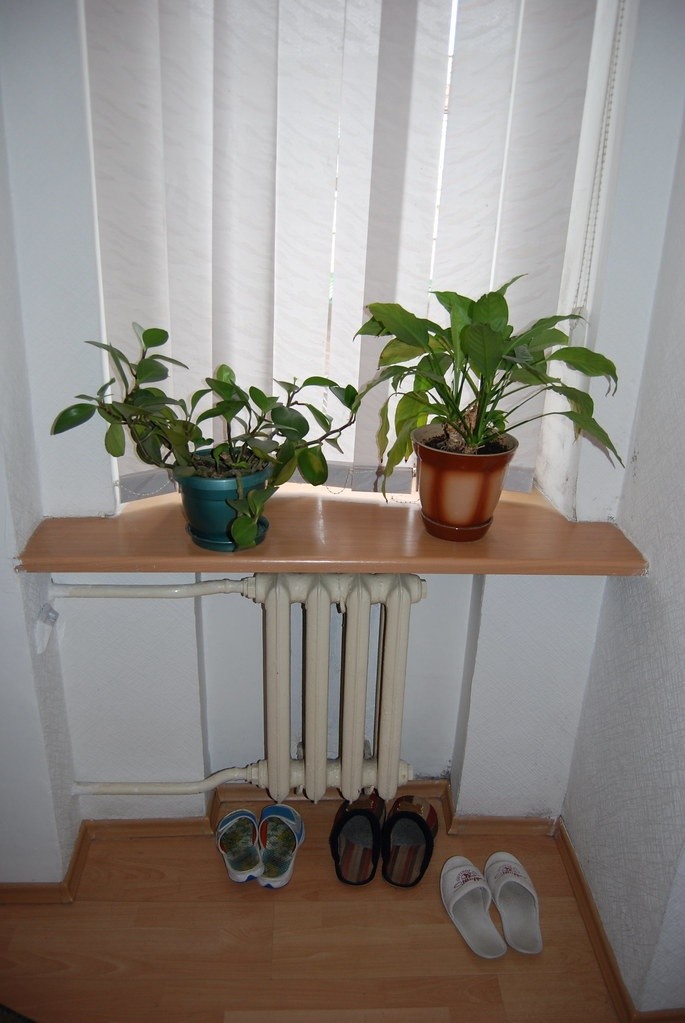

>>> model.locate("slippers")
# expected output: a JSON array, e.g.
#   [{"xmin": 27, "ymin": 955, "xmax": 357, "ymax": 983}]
[
  {"xmin": 484, "ymin": 853, "xmax": 543, "ymax": 955},
  {"xmin": 328, "ymin": 794, "xmax": 386, "ymax": 885},
  {"xmin": 439, "ymin": 856, "xmax": 507, "ymax": 958},
  {"xmin": 258, "ymin": 804, "xmax": 304, "ymax": 888},
  {"xmin": 380, "ymin": 795, "xmax": 439, "ymax": 888},
  {"xmin": 216, "ymin": 809, "xmax": 264, "ymax": 882}
]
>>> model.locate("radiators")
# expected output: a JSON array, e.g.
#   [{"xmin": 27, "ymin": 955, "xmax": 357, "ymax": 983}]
[{"xmin": 232, "ymin": 571, "xmax": 425, "ymax": 803}]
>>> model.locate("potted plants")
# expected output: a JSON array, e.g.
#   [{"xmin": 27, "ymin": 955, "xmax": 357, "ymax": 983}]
[
  {"xmin": 351, "ymin": 273, "xmax": 627, "ymax": 539},
  {"xmin": 51, "ymin": 319, "xmax": 360, "ymax": 553}
]
